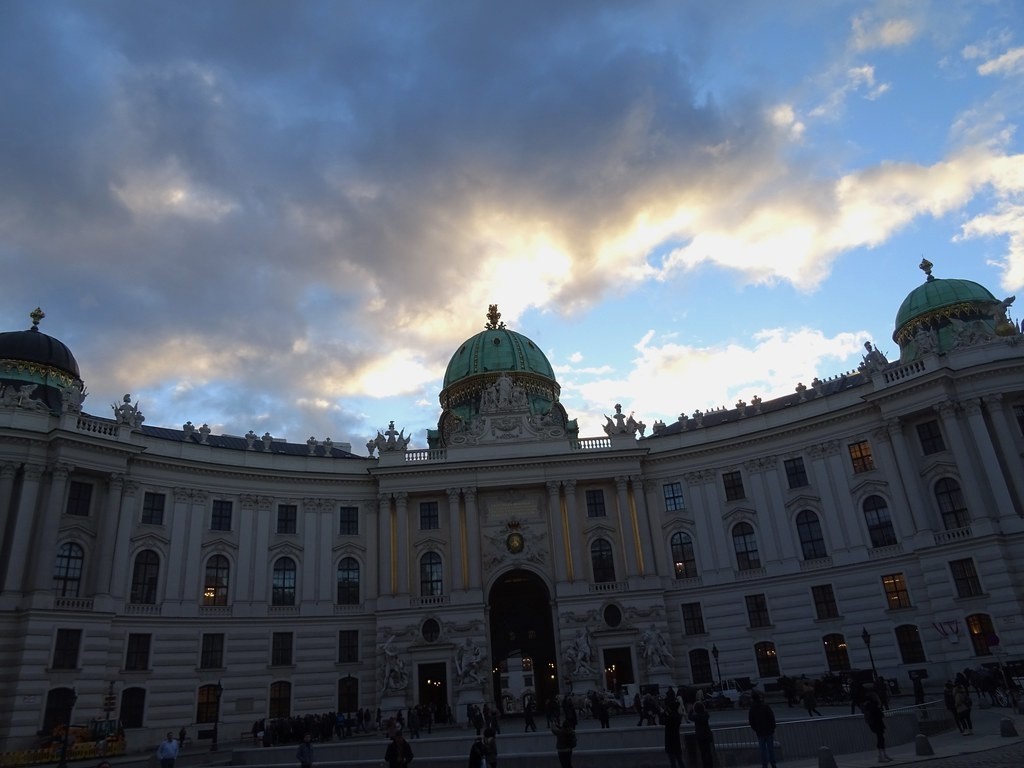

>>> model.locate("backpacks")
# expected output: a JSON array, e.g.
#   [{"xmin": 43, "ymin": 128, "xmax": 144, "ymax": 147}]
[
  {"xmin": 564, "ymin": 728, "xmax": 578, "ymax": 749},
  {"xmin": 961, "ymin": 695, "xmax": 973, "ymax": 705}
]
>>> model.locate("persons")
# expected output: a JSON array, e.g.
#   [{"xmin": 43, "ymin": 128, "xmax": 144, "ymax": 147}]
[
  {"xmin": 251, "ymin": 701, "xmax": 453, "ymax": 747},
  {"xmin": 179, "ymin": 728, "xmax": 186, "ymax": 748},
  {"xmin": 483, "ymin": 372, "xmax": 527, "ymax": 409},
  {"xmin": 466, "ymin": 703, "xmax": 501, "ymax": 768},
  {"xmin": 566, "ymin": 626, "xmax": 599, "ymax": 675},
  {"xmin": 944, "ymin": 667, "xmax": 985, "ymax": 736},
  {"xmin": 687, "ymin": 702, "xmax": 713, "ymax": 768},
  {"xmin": 379, "ymin": 643, "xmax": 409, "ymax": 692},
  {"xmin": 549, "ymin": 720, "xmax": 573, "ymax": 768},
  {"xmin": 524, "ymin": 685, "xmax": 760, "ymax": 732},
  {"xmin": 659, "ymin": 703, "xmax": 685, "ymax": 768},
  {"xmin": 779, "ymin": 670, "xmax": 929, "ymax": 719},
  {"xmin": 859, "ymin": 694, "xmax": 893, "ymax": 762},
  {"xmin": 385, "ymin": 730, "xmax": 414, "ymax": 768},
  {"xmin": 456, "ymin": 637, "xmax": 481, "ymax": 686},
  {"xmin": 641, "ymin": 623, "xmax": 671, "ymax": 670},
  {"xmin": 97, "ymin": 761, "xmax": 111, "ymax": 768},
  {"xmin": 156, "ymin": 732, "xmax": 179, "ymax": 768},
  {"xmin": 749, "ymin": 694, "xmax": 776, "ymax": 768},
  {"xmin": 296, "ymin": 734, "xmax": 314, "ymax": 768}
]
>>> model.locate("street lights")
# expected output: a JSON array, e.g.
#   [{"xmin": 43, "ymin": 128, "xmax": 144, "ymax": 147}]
[
  {"xmin": 210, "ymin": 680, "xmax": 224, "ymax": 751},
  {"xmin": 345, "ymin": 672, "xmax": 353, "ymax": 737},
  {"xmin": 57, "ymin": 687, "xmax": 77, "ymax": 768},
  {"xmin": 861, "ymin": 626, "xmax": 880, "ymax": 682},
  {"xmin": 712, "ymin": 643, "xmax": 721, "ymax": 685}
]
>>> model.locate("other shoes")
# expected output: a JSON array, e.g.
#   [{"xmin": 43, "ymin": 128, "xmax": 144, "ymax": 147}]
[{"xmin": 878, "ymin": 756, "xmax": 894, "ymax": 764}]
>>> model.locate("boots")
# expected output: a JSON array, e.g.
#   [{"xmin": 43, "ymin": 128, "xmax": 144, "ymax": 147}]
[{"xmin": 963, "ymin": 727, "xmax": 975, "ymax": 736}]
